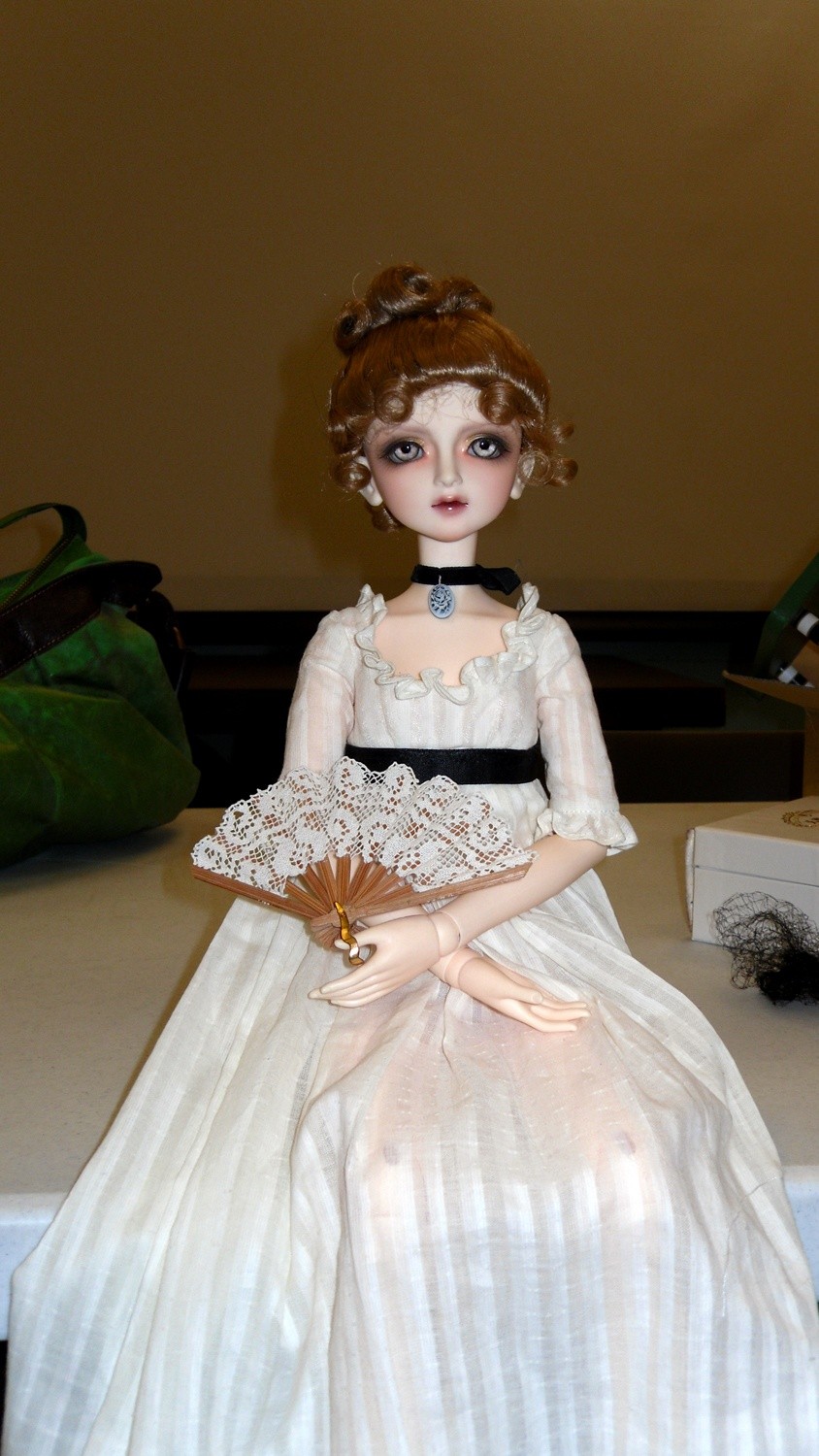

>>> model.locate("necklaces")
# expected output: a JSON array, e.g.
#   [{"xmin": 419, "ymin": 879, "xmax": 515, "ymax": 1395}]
[{"xmin": 409, "ymin": 562, "xmax": 517, "ymax": 618}]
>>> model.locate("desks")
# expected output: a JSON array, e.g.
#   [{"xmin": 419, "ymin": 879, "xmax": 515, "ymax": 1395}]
[{"xmin": 0, "ymin": 802, "xmax": 818, "ymax": 1338}]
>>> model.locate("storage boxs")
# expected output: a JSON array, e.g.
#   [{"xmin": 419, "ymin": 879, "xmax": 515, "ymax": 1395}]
[
  {"xmin": 722, "ymin": 640, "xmax": 819, "ymax": 797},
  {"xmin": 684, "ymin": 802, "xmax": 819, "ymax": 953}
]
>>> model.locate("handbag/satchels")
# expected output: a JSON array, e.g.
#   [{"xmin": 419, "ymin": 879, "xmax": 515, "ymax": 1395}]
[{"xmin": 0, "ymin": 499, "xmax": 203, "ymax": 873}]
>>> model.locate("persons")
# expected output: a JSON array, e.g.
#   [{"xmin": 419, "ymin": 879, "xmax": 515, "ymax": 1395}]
[{"xmin": 6, "ymin": 263, "xmax": 819, "ymax": 1455}]
